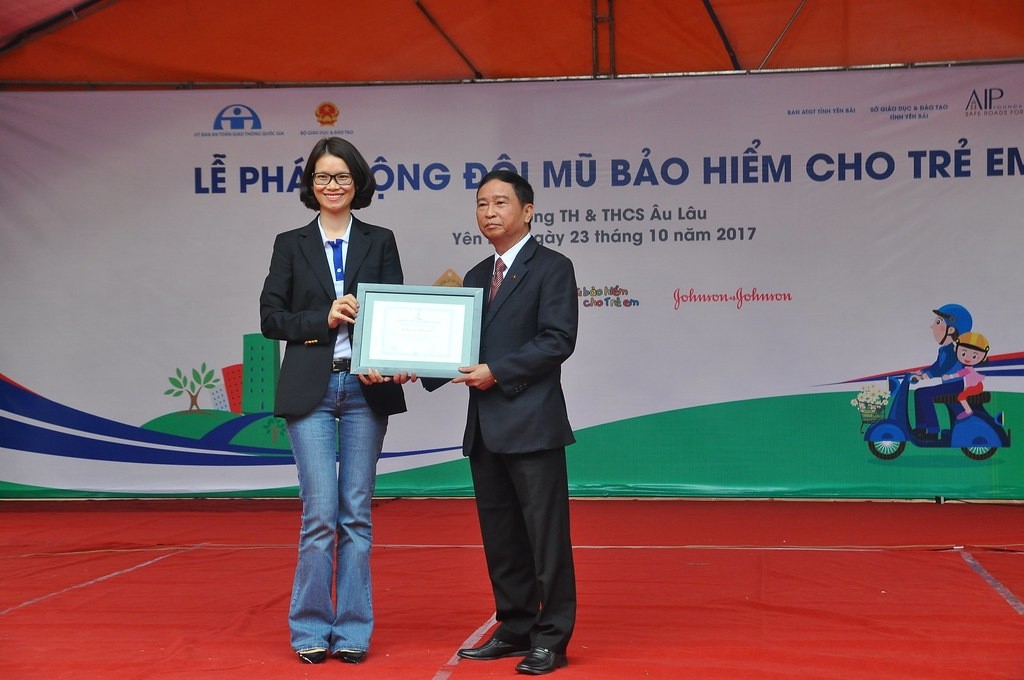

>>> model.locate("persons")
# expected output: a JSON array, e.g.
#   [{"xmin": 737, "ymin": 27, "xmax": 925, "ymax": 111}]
[
  {"xmin": 393, "ymin": 170, "xmax": 578, "ymax": 676},
  {"xmin": 259, "ymin": 137, "xmax": 407, "ymax": 664}
]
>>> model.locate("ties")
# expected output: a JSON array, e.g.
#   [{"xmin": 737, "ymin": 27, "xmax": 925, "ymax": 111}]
[{"xmin": 488, "ymin": 256, "xmax": 507, "ymax": 310}]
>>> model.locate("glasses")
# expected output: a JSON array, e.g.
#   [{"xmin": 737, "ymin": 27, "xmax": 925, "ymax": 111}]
[{"xmin": 311, "ymin": 171, "xmax": 355, "ymax": 185}]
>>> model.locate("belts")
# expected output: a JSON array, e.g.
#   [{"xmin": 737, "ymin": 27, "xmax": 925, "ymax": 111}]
[{"xmin": 331, "ymin": 359, "xmax": 352, "ymax": 373}]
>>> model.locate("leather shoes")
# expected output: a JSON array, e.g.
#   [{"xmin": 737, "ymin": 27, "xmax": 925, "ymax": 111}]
[
  {"xmin": 515, "ymin": 646, "xmax": 567, "ymax": 675},
  {"xmin": 457, "ymin": 635, "xmax": 532, "ymax": 660}
]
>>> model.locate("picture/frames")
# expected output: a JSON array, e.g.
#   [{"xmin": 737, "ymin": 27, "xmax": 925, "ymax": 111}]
[{"xmin": 350, "ymin": 283, "xmax": 484, "ymax": 378}]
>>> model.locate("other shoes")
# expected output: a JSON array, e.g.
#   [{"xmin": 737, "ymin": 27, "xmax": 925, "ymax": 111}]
[
  {"xmin": 299, "ymin": 651, "xmax": 326, "ymax": 663},
  {"xmin": 338, "ymin": 651, "xmax": 366, "ymax": 664}
]
